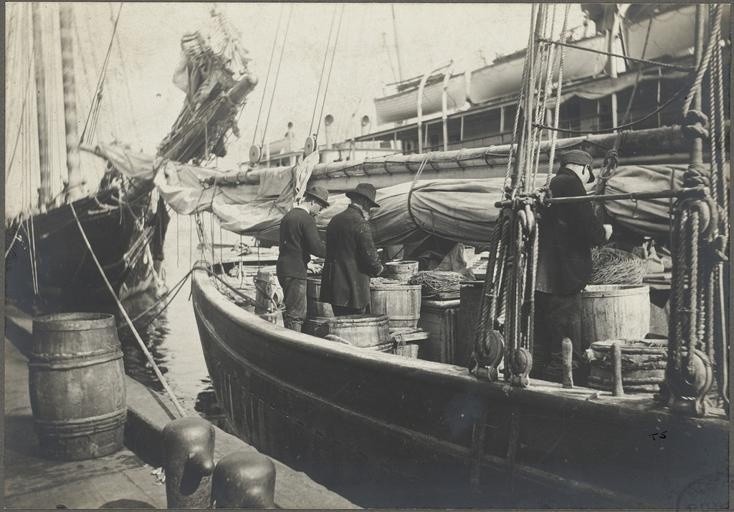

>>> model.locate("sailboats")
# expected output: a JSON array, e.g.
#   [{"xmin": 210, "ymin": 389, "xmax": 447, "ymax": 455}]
[
  {"xmin": 1, "ymin": 2, "xmax": 262, "ymax": 319},
  {"xmin": 188, "ymin": 1, "xmax": 733, "ymax": 509}
]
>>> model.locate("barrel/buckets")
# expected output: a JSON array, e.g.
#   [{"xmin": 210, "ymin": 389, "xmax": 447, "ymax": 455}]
[
  {"xmin": 29, "ymin": 311, "xmax": 124, "ymax": 461},
  {"xmin": 256, "ymin": 258, "xmax": 428, "ymax": 362},
  {"xmin": 580, "ymin": 283, "xmax": 649, "ymax": 343}
]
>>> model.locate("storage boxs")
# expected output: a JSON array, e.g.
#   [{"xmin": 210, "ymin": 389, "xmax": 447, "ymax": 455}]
[
  {"xmin": 420, "ymin": 300, "xmax": 461, "ymax": 364},
  {"xmin": 255, "ymin": 265, "xmax": 285, "ymax": 328}
]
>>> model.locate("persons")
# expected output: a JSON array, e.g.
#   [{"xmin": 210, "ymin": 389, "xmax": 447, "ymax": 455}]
[
  {"xmin": 531, "ymin": 150, "xmax": 613, "ymax": 381},
  {"xmin": 318, "ymin": 183, "xmax": 383, "ymax": 317},
  {"xmin": 274, "ymin": 185, "xmax": 329, "ymax": 333}
]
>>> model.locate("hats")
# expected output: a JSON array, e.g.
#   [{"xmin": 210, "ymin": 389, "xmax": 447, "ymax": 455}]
[
  {"xmin": 304, "ymin": 185, "xmax": 330, "ymax": 206},
  {"xmin": 560, "ymin": 149, "xmax": 595, "ymax": 183},
  {"xmin": 345, "ymin": 182, "xmax": 380, "ymax": 207}
]
